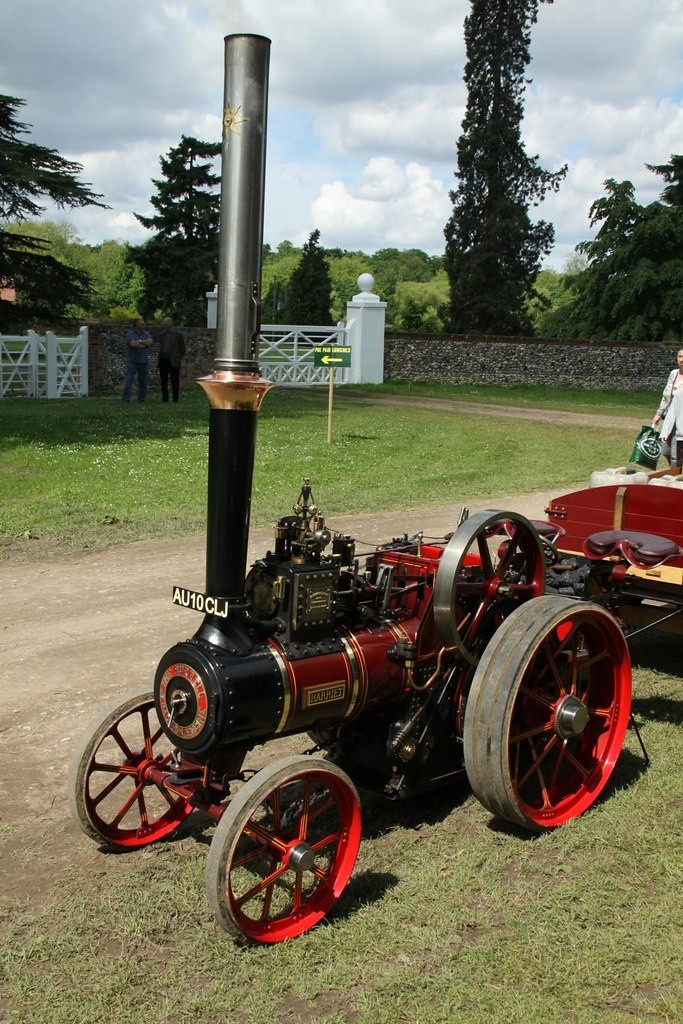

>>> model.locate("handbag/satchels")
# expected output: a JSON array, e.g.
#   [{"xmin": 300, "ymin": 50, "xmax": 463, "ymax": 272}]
[
  {"xmin": 660, "ymin": 395, "xmax": 674, "ymax": 419},
  {"xmin": 628, "ymin": 423, "xmax": 663, "ymax": 471}
]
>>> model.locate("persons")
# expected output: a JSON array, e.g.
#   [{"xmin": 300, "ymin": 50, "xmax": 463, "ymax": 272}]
[
  {"xmin": 651, "ymin": 348, "xmax": 683, "ymax": 469},
  {"xmin": 121, "ymin": 318, "xmax": 153, "ymax": 402},
  {"xmin": 156, "ymin": 318, "xmax": 185, "ymax": 403}
]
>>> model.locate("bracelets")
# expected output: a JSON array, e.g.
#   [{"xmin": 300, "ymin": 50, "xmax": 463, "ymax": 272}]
[{"xmin": 139, "ymin": 340, "xmax": 142, "ymax": 344}]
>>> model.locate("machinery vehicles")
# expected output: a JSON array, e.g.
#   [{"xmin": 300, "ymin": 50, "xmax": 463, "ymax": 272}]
[{"xmin": 74, "ymin": 33, "xmax": 683, "ymax": 950}]
